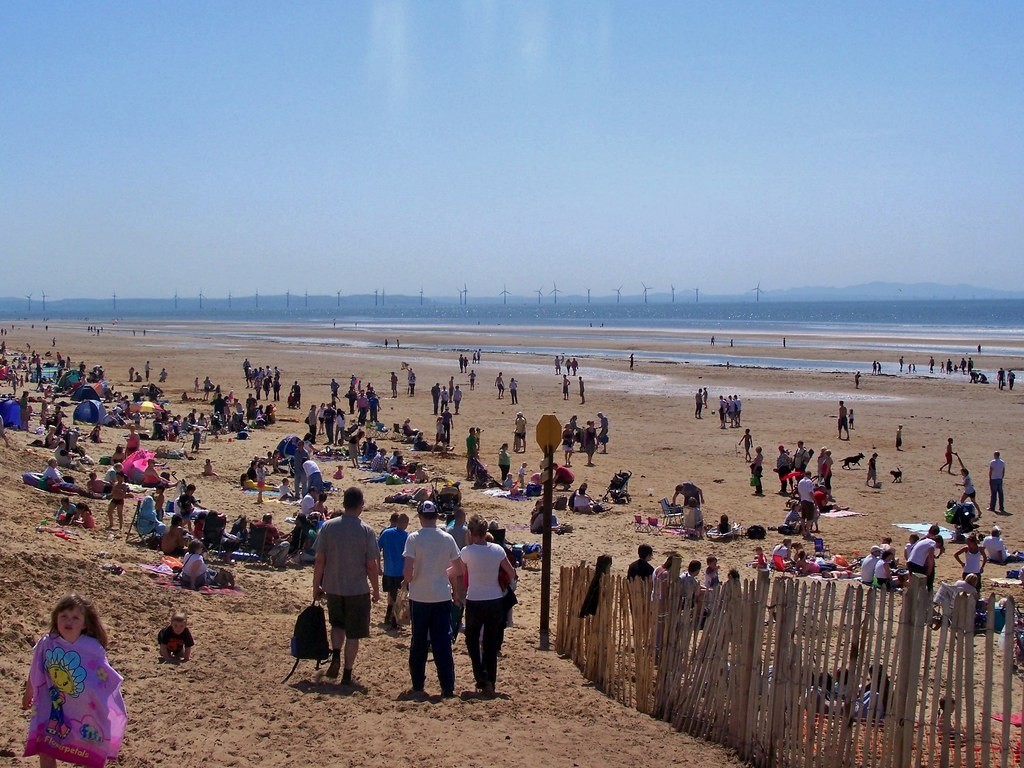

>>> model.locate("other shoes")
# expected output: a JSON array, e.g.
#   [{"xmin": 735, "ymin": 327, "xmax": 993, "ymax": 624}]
[
  {"xmin": 333, "ymin": 652, "xmax": 341, "ymax": 664},
  {"xmin": 342, "ymin": 672, "xmax": 352, "ymax": 684}
]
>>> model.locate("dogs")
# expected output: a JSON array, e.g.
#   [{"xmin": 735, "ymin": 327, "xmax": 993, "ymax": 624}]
[
  {"xmin": 838, "ymin": 452, "xmax": 865, "ymax": 470},
  {"xmin": 890, "ymin": 468, "xmax": 902, "ymax": 483}
]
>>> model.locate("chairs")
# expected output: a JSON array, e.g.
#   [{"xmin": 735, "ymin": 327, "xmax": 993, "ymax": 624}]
[
  {"xmin": 813, "ymin": 538, "xmax": 831, "ymax": 559},
  {"xmin": 243, "ymin": 521, "xmax": 273, "ymax": 567},
  {"xmin": 202, "ymin": 511, "xmax": 228, "ymax": 558},
  {"xmin": 435, "ymin": 493, "xmax": 460, "ymax": 517},
  {"xmin": 126, "ymin": 500, "xmax": 157, "ymax": 547},
  {"xmin": 634, "ymin": 496, "xmax": 684, "ymax": 535}
]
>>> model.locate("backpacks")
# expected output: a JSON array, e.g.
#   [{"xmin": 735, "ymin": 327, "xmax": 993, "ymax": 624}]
[{"xmin": 291, "ymin": 598, "xmax": 332, "ymax": 660}]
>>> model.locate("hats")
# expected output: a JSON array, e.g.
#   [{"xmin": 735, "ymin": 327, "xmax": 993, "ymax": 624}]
[
  {"xmin": 870, "ymin": 546, "xmax": 881, "ymax": 552},
  {"xmin": 416, "ymin": 500, "xmax": 439, "ymax": 514},
  {"xmin": 113, "ymin": 463, "xmax": 124, "ymax": 469},
  {"xmin": 517, "ymin": 412, "xmax": 523, "ymax": 416},
  {"xmin": 779, "ymin": 445, "xmax": 784, "ymax": 449}
]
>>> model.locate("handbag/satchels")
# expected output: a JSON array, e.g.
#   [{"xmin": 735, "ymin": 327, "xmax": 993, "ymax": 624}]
[
  {"xmin": 305, "ymin": 417, "xmax": 310, "ymax": 423},
  {"xmin": 750, "ymin": 474, "xmax": 756, "ymax": 486},
  {"xmin": 504, "ymin": 586, "xmax": 518, "ymax": 612},
  {"xmin": 599, "ymin": 435, "xmax": 610, "ymax": 443},
  {"xmin": 394, "ymin": 584, "xmax": 411, "ymax": 625}
]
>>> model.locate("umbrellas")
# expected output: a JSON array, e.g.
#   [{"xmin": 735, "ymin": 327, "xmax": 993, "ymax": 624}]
[
  {"xmin": 53, "ymin": 369, "xmax": 83, "ymax": 393},
  {"xmin": 123, "ymin": 398, "xmax": 164, "ymax": 440}
]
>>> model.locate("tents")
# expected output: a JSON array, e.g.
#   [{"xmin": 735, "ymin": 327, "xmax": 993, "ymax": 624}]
[
  {"xmin": 70, "ymin": 384, "xmax": 101, "ymax": 402},
  {"xmin": 1, "ymin": 397, "xmax": 27, "ymax": 429},
  {"xmin": 73, "ymin": 399, "xmax": 106, "ymax": 426},
  {"xmin": 276, "ymin": 435, "xmax": 305, "ymax": 459}
]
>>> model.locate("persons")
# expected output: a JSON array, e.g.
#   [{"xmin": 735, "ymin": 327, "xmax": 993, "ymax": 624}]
[
  {"xmin": 450, "ymin": 514, "xmax": 517, "ymax": 697},
  {"xmin": 159, "ymin": 612, "xmax": 195, "ymax": 661},
  {"xmin": 0, "ymin": 323, "xmax": 1023, "ymax": 721},
  {"xmin": 311, "ymin": 486, "xmax": 380, "ymax": 696},
  {"xmin": 400, "ymin": 500, "xmax": 464, "ymax": 699},
  {"xmin": 23, "ymin": 594, "xmax": 108, "ymax": 768}
]
